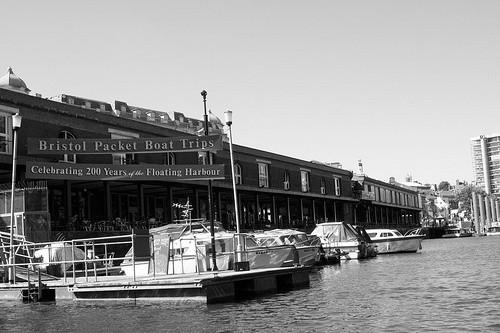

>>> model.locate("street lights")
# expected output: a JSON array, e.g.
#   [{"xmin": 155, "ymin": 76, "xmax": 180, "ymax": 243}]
[
  {"xmin": 222, "ymin": 108, "xmax": 251, "ymax": 270},
  {"xmin": 8, "ymin": 110, "xmax": 23, "ymax": 284}
]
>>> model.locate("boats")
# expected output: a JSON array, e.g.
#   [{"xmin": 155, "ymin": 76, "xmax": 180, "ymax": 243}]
[
  {"xmin": 308, "ymin": 221, "xmax": 380, "ymax": 261},
  {"xmin": 358, "ymin": 227, "xmax": 426, "ymax": 255},
  {"xmin": 27, "ymin": 240, "xmax": 126, "ymax": 278},
  {"xmin": 119, "ymin": 197, "xmax": 293, "ymax": 276},
  {"xmin": 442, "ymin": 228, "xmax": 475, "ymax": 237},
  {"xmin": 479, "ymin": 227, "xmax": 500, "ymax": 236},
  {"xmin": 249, "ymin": 227, "xmax": 322, "ymax": 270}
]
